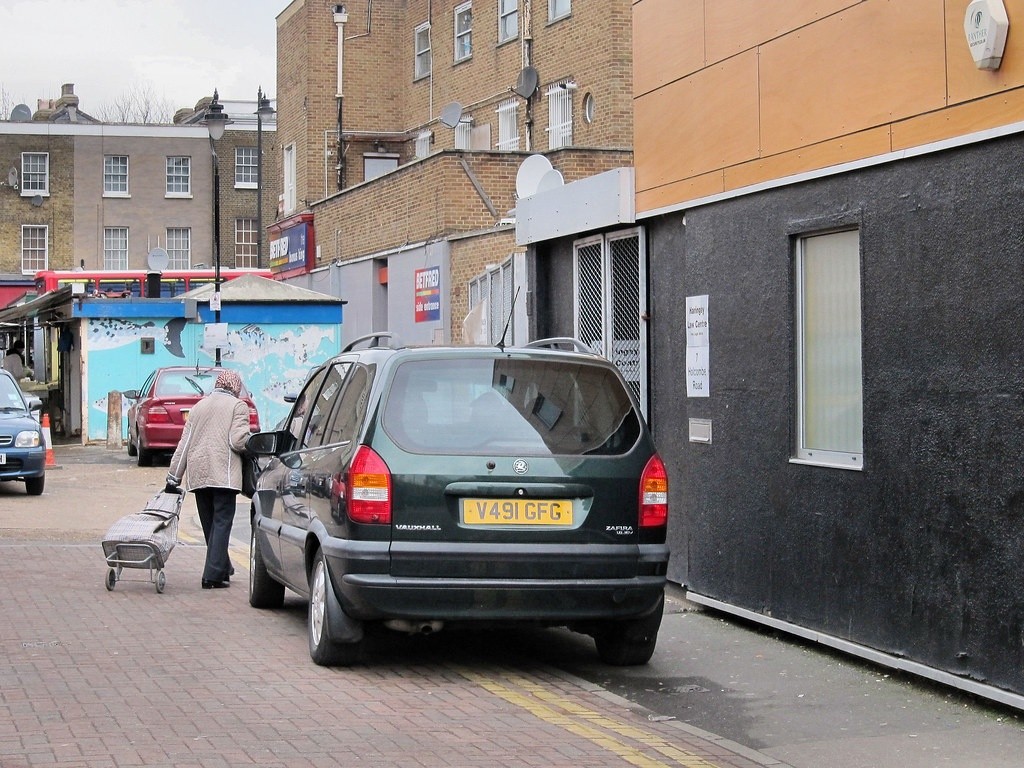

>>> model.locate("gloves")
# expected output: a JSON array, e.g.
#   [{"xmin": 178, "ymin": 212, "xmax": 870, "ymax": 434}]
[{"xmin": 166, "ymin": 483, "xmax": 176, "ymax": 492}]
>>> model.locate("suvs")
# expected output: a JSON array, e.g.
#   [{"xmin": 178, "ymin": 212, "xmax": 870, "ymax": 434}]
[{"xmin": 246, "ymin": 330, "xmax": 670, "ymax": 669}]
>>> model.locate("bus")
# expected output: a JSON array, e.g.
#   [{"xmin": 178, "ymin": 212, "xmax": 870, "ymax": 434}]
[{"xmin": 36, "ymin": 269, "xmax": 275, "ymax": 299}]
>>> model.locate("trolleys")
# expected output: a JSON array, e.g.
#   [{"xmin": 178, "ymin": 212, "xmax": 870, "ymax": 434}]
[{"xmin": 101, "ymin": 487, "xmax": 188, "ymax": 593}]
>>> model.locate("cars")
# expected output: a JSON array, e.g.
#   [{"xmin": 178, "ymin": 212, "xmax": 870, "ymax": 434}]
[
  {"xmin": 123, "ymin": 368, "xmax": 260, "ymax": 467},
  {"xmin": 0, "ymin": 369, "xmax": 46, "ymax": 495}
]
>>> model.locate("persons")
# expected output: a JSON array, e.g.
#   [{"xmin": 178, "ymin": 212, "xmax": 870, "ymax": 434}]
[
  {"xmin": 165, "ymin": 369, "xmax": 256, "ymax": 589},
  {"xmin": 3, "ymin": 340, "xmax": 29, "ymax": 385}
]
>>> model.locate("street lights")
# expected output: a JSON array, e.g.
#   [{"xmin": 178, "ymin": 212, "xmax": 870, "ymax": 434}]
[
  {"xmin": 254, "ymin": 86, "xmax": 276, "ymax": 270},
  {"xmin": 200, "ymin": 89, "xmax": 237, "ymax": 367}
]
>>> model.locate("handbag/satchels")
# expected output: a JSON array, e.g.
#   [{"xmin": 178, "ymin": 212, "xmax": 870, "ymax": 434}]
[{"xmin": 240, "ymin": 451, "xmax": 263, "ymax": 498}]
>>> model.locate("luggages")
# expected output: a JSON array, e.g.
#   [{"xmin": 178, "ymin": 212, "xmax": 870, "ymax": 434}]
[{"xmin": 101, "ymin": 487, "xmax": 186, "ymax": 593}]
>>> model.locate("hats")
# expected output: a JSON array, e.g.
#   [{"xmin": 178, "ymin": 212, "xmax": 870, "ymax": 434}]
[{"xmin": 14, "ymin": 340, "xmax": 25, "ymax": 349}]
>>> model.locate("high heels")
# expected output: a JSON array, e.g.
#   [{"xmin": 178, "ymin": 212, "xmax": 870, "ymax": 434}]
[
  {"xmin": 230, "ymin": 568, "xmax": 235, "ymax": 575},
  {"xmin": 202, "ymin": 577, "xmax": 230, "ymax": 588}
]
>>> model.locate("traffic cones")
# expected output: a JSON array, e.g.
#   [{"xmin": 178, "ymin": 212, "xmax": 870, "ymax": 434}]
[{"xmin": 41, "ymin": 414, "xmax": 63, "ymax": 471}]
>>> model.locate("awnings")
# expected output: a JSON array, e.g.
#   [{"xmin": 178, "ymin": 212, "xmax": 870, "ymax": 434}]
[{"xmin": 0, "ymin": 291, "xmax": 61, "ymax": 322}]
